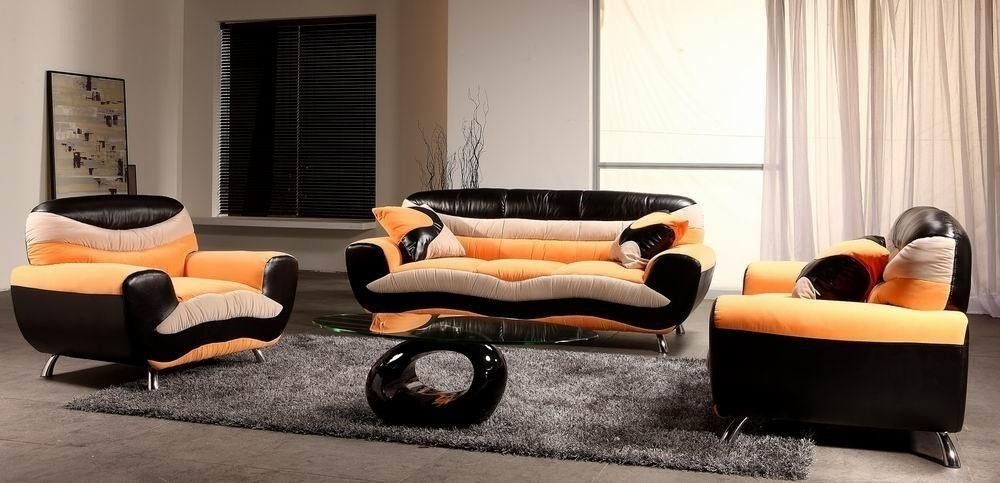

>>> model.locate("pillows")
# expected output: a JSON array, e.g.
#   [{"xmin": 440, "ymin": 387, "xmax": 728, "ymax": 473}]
[
  {"xmin": 609, "ymin": 210, "xmax": 689, "ymax": 269},
  {"xmin": 372, "ymin": 204, "xmax": 466, "ymax": 262},
  {"xmin": 792, "ymin": 235, "xmax": 890, "ymax": 302}
]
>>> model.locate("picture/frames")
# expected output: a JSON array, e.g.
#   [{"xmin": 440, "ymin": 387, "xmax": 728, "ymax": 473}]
[{"xmin": 46, "ymin": 70, "xmax": 130, "ymax": 199}]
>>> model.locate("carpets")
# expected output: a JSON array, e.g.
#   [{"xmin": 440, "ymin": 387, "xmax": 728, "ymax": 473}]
[{"xmin": 63, "ymin": 334, "xmax": 815, "ymax": 480}]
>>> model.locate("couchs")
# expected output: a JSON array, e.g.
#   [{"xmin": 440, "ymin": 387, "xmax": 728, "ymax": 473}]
[
  {"xmin": 345, "ymin": 188, "xmax": 715, "ymax": 354},
  {"xmin": 11, "ymin": 195, "xmax": 299, "ymax": 393},
  {"xmin": 708, "ymin": 207, "xmax": 972, "ymax": 469}
]
